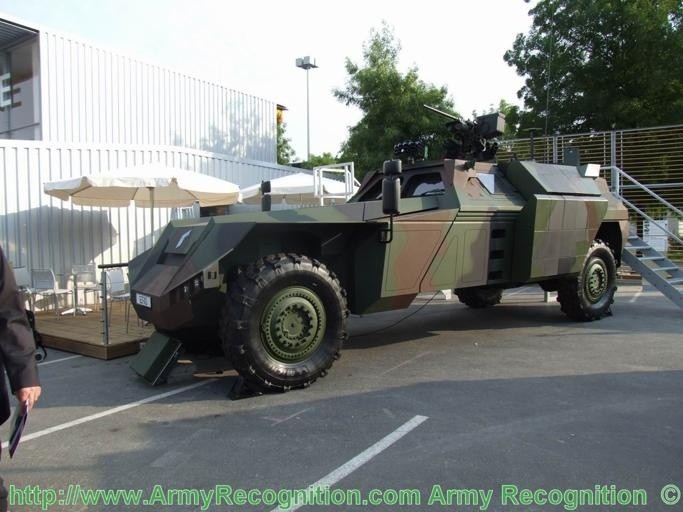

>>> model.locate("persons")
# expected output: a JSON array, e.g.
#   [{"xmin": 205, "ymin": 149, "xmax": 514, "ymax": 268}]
[{"xmin": 0, "ymin": 243, "xmax": 43, "ymax": 511}]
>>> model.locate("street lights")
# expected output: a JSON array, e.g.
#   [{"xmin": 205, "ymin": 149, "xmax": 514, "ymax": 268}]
[
  {"xmin": 524, "ymin": 0, "xmax": 554, "ymax": 136},
  {"xmin": 295, "ymin": 55, "xmax": 319, "ymax": 163}
]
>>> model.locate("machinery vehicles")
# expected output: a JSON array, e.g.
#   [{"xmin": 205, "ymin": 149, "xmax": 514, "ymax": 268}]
[{"xmin": 127, "ymin": 102, "xmax": 629, "ymax": 401}]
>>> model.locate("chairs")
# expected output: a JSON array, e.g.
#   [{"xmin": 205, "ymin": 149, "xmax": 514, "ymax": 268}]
[{"xmin": 12, "ymin": 264, "xmax": 144, "ymax": 335}]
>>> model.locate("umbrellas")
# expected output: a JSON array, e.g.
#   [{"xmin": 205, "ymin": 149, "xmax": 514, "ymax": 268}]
[
  {"xmin": 43, "ymin": 161, "xmax": 241, "ymax": 246},
  {"xmin": 240, "ymin": 169, "xmax": 357, "ymax": 207}
]
